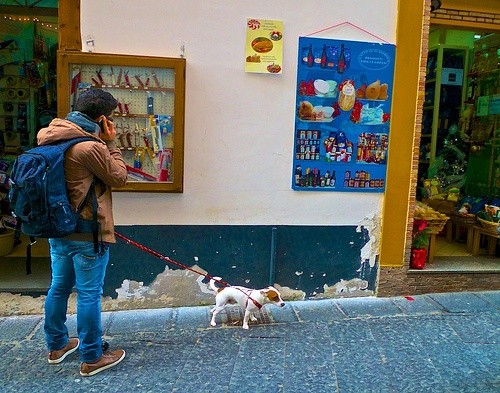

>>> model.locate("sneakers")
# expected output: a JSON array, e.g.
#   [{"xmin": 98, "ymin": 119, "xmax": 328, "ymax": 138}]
[
  {"xmin": 80, "ymin": 350, "xmax": 125, "ymax": 376},
  {"xmin": 48, "ymin": 338, "xmax": 80, "ymax": 363}
]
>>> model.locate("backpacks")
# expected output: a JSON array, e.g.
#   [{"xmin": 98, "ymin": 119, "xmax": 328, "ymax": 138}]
[{"xmin": 9, "ymin": 137, "xmax": 106, "ymax": 237}]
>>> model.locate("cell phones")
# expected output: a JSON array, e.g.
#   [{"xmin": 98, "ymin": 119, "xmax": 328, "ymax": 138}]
[{"xmin": 98, "ymin": 118, "xmax": 105, "ymax": 132}]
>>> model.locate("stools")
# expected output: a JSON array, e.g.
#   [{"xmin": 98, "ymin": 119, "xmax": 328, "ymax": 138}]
[{"xmin": 413, "ymin": 214, "xmax": 500, "ymax": 263}]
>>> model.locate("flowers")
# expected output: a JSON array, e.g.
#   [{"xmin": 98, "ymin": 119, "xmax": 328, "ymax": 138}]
[{"xmin": 413, "ymin": 221, "xmax": 429, "ymax": 247}]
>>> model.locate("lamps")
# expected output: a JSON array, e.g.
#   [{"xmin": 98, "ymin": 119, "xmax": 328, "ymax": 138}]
[{"xmin": 473, "ymin": 32, "xmax": 481, "ymax": 38}]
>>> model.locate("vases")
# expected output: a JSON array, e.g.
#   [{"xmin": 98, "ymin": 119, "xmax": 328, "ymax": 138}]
[{"xmin": 412, "ymin": 249, "xmax": 428, "ymax": 269}]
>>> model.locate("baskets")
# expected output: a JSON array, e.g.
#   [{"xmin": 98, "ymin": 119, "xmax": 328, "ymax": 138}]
[
  {"xmin": 414, "ymin": 212, "xmax": 450, "ymax": 232},
  {"xmin": 422, "ymin": 195, "xmax": 461, "ymax": 214},
  {"xmin": 458, "ymin": 213, "xmax": 478, "ymax": 224},
  {"xmin": 478, "ymin": 214, "xmax": 500, "ymax": 234}
]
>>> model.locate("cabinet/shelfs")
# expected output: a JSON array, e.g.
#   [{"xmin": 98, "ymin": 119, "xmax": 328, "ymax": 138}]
[{"xmin": 419, "ymin": 45, "xmax": 470, "ymax": 168}]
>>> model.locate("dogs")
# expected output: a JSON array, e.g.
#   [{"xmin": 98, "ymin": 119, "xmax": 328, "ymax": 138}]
[{"xmin": 210, "ymin": 276, "xmax": 285, "ymax": 331}]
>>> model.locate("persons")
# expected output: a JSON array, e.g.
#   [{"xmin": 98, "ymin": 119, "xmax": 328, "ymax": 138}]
[{"xmin": 35, "ymin": 87, "xmax": 129, "ymax": 378}]
